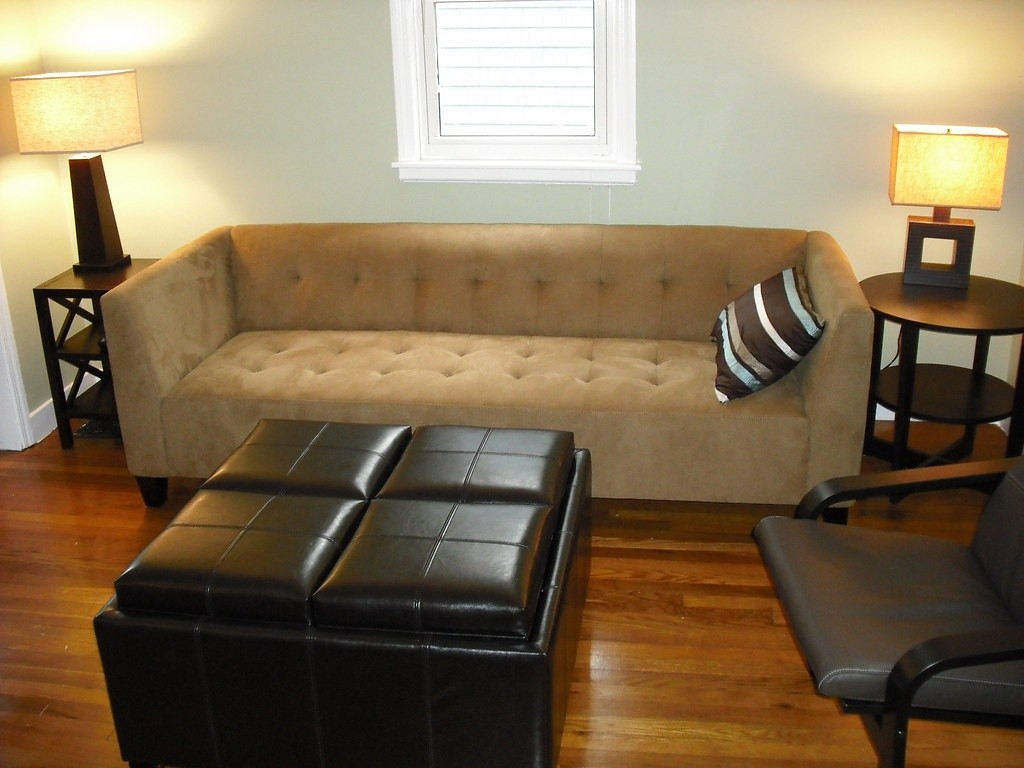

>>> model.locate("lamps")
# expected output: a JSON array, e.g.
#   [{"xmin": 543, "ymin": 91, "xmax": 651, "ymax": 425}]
[
  {"xmin": 888, "ymin": 124, "xmax": 1010, "ymax": 289},
  {"xmin": 9, "ymin": 69, "xmax": 142, "ymax": 274}
]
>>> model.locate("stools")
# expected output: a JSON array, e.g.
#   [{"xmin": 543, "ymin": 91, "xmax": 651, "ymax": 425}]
[{"xmin": 90, "ymin": 417, "xmax": 593, "ymax": 768}]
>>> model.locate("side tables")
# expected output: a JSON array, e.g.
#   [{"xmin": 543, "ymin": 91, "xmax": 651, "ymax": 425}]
[
  {"xmin": 31, "ymin": 257, "xmax": 160, "ymax": 448},
  {"xmin": 857, "ymin": 271, "xmax": 1024, "ymax": 501}
]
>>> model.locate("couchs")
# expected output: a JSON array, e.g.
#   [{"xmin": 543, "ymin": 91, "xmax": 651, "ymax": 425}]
[{"xmin": 99, "ymin": 223, "xmax": 876, "ymax": 527}]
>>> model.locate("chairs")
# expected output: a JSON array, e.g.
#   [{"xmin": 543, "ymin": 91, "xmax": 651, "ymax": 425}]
[{"xmin": 750, "ymin": 448, "xmax": 1024, "ymax": 768}]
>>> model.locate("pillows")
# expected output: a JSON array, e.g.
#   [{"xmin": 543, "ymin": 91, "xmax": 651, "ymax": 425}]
[{"xmin": 708, "ymin": 266, "xmax": 828, "ymax": 405}]
